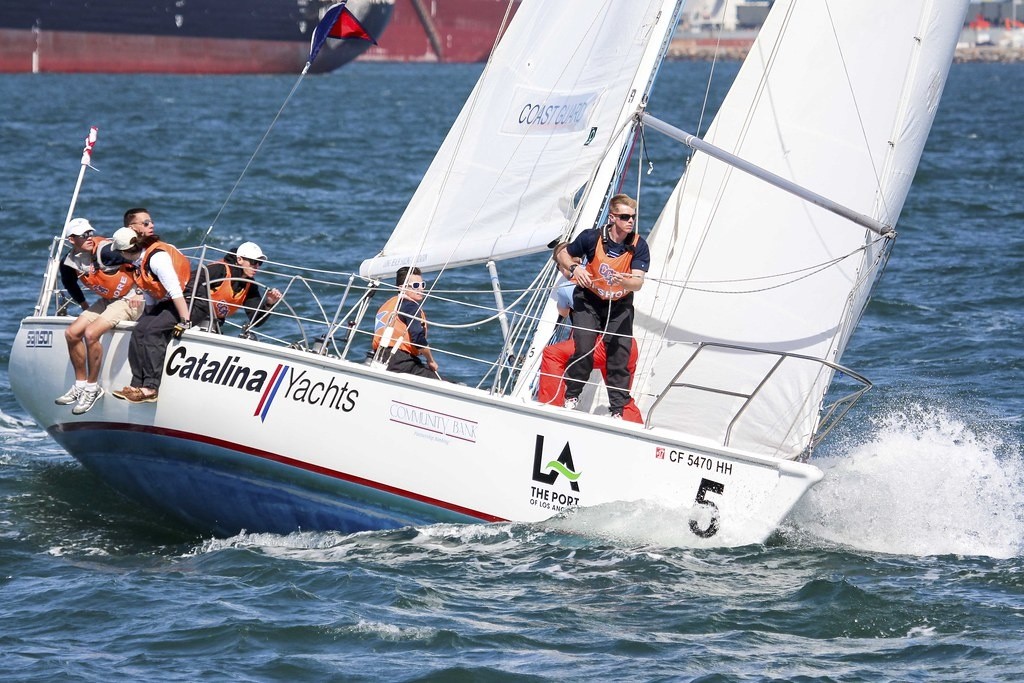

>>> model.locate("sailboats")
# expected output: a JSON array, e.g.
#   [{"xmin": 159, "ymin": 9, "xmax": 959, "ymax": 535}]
[{"xmin": 9, "ymin": 1, "xmax": 972, "ymax": 554}]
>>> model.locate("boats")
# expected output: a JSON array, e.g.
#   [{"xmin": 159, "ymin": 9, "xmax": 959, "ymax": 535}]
[{"xmin": 0, "ymin": 0, "xmax": 394, "ymax": 74}]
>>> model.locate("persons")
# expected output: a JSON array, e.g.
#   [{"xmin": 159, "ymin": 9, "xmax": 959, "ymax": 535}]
[
  {"xmin": 54, "ymin": 218, "xmax": 144, "ymax": 415},
  {"xmin": 111, "ymin": 227, "xmax": 208, "ymax": 403},
  {"xmin": 538, "ymin": 242, "xmax": 644, "ymax": 424},
  {"xmin": 194, "ymin": 241, "xmax": 283, "ymax": 327},
  {"xmin": 554, "ymin": 194, "xmax": 650, "ymax": 419},
  {"xmin": 372, "ymin": 267, "xmax": 460, "ymax": 383},
  {"xmin": 124, "ymin": 208, "xmax": 154, "ymax": 236}
]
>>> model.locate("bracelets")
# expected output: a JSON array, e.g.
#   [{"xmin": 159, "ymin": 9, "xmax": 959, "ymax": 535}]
[
  {"xmin": 266, "ymin": 303, "xmax": 273, "ymax": 307},
  {"xmin": 186, "ymin": 320, "xmax": 190, "ymax": 322}
]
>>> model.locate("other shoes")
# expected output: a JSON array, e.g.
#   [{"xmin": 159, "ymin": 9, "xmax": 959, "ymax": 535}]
[
  {"xmin": 123, "ymin": 387, "xmax": 158, "ymax": 403},
  {"xmin": 561, "ymin": 397, "xmax": 579, "ymax": 409},
  {"xmin": 112, "ymin": 386, "xmax": 137, "ymax": 399},
  {"xmin": 611, "ymin": 413, "xmax": 622, "ymax": 420}
]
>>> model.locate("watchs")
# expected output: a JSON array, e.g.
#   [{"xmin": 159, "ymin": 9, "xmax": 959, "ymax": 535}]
[
  {"xmin": 569, "ymin": 264, "xmax": 577, "ymax": 272},
  {"xmin": 135, "ymin": 290, "xmax": 143, "ymax": 295}
]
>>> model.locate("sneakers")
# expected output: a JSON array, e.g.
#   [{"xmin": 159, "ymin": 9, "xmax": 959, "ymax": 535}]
[
  {"xmin": 72, "ymin": 385, "xmax": 105, "ymax": 415},
  {"xmin": 54, "ymin": 384, "xmax": 86, "ymax": 405}
]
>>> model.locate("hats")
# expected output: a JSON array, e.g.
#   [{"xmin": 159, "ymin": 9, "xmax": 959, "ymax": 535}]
[
  {"xmin": 67, "ymin": 218, "xmax": 96, "ymax": 237},
  {"xmin": 236, "ymin": 242, "xmax": 267, "ymax": 261},
  {"xmin": 110, "ymin": 227, "xmax": 138, "ymax": 252}
]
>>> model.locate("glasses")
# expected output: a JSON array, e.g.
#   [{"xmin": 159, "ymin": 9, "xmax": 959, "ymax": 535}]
[
  {"xmin": 73, "ymin": 230, "xmax": 93, "ymax": 239},
  {"xmin": 405, "ymin": 281, "xmax": 426, "ymax": 290},
  {"xmin": 131, "ymin": 220, "xmax": 154, "ymax": 227},
  {"xmin": 611, "ymin": 214, "xmax": 636, "ymax": 221},
  {"xmin": 241, "ymin": 257, "xmax": 263, "ymax": 266}
]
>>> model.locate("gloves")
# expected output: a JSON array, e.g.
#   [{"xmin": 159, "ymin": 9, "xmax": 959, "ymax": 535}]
[{"xmin": 172, "ymin": 317, "xmax": 190, "ymax": 340}]
